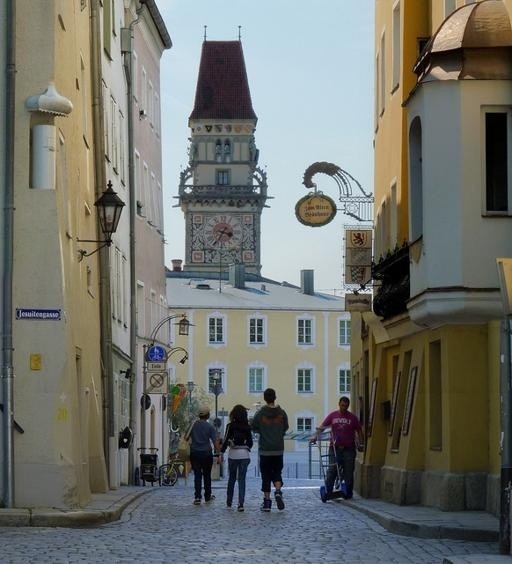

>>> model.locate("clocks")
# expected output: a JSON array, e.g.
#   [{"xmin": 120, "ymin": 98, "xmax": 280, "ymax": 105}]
[{"xmin": 203, "ymin": 215, "xmax": 242, "ymax": 249}]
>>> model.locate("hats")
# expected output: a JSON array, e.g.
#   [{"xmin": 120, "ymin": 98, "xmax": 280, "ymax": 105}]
[{"xmin": 198, "ymin": 406, "xmax": 209, "ymax": 415}]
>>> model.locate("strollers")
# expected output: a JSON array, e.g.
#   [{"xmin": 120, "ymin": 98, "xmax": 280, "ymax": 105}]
[{"xmin": 138, "ymin": 447, "xmax": 160, "ymax": 486}]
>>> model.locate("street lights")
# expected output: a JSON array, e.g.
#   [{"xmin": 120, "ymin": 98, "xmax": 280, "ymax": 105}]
[
  {"xmin": 208, "ymin": 367, "xmax": 223, "ymax": 420},
  {"xmin": 187, "ymin": 379, "xmax": 194, "ymax": 411}
]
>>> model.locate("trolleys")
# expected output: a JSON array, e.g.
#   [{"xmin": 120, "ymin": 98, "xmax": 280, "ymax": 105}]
[{"xmin": 311, "ymin": 429, "xmax": 353, "ymax": 503}]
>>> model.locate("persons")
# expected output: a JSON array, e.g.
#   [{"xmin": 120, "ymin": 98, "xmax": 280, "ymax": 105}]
[
  {"xmin": 309, "ymin": 397, "xmax": 363, "ymax": 499},
  {"xmin": 253, "ymin": 389, "xmax": 289, "ymax": 512},
  {"xmin": 217, "ymin": 405, "xmax": 253, "ymax": 511},
  {"xmin": 183, "ymin": 406, "xmax": 222, "ymax": 505}
]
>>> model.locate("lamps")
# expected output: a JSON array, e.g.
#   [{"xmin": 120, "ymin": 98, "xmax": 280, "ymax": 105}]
[
  {"xmin": 146, "ymin": 313, "xmax": 196, "ymax": 349},
  {"xmin": 77, "ymin": 181, "xmax": 126, "ymax": 261},
  {"xmin": 168, "ymin": 346, "xmax": 189, "ymax": 366}
]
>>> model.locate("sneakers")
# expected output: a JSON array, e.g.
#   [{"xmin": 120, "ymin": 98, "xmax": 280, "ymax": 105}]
[
  {"xmin": 206, "ymin": 495, "xmax": 215, "ymax": 503},
  {"xmin": 238, "ymin": 506, "xmax": 244, "ymax": 511},
  {"xmin": 260, "ymin": 499, "xmax": 272, "ymax": 511},
  {"xmin": 194, "ymin": 499, "xmax": 200, "ymax": 505},
  {"xmin": 274, "ymin": 491, "xmax": 284, "ymax": 510}
]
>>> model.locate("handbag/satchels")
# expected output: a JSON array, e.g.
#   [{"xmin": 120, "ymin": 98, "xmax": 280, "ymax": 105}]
[{"xmin": 177, "ymin": 439, "xmax": 190, "ymax": 457}]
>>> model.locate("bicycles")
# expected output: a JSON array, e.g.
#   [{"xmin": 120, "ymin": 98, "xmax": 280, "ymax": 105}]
[{"xmin": 159, "ymin": 446, "xmax": 185, "ymax": 485}]
